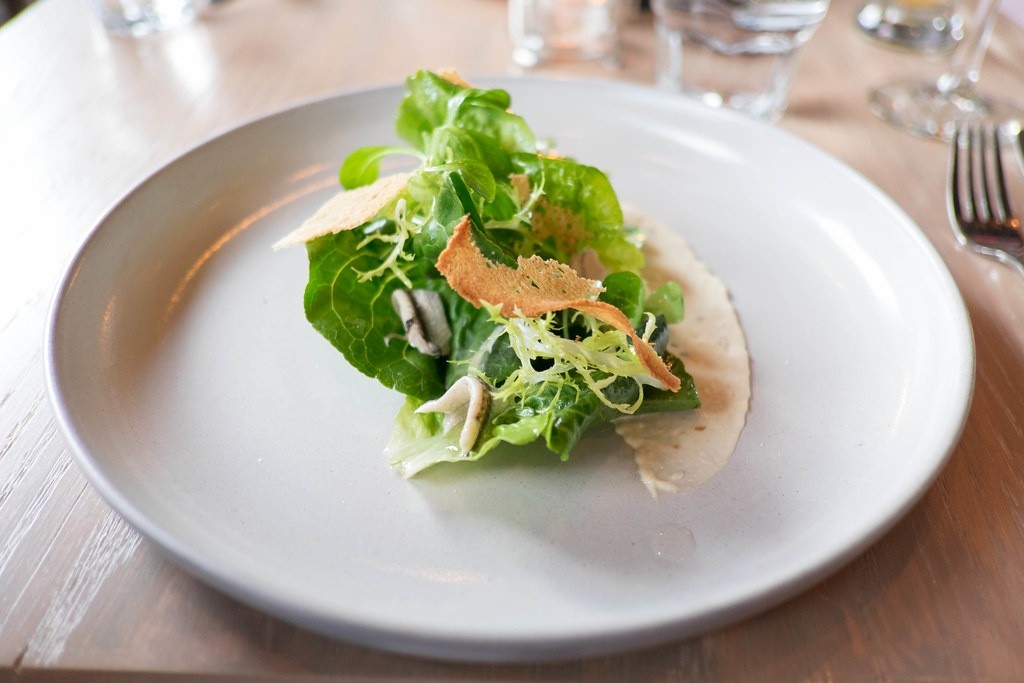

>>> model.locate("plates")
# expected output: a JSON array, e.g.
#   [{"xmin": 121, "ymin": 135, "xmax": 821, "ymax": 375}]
[{"xmin": 43, "ymin": 68, "xmax": 978, "ymax": 667}]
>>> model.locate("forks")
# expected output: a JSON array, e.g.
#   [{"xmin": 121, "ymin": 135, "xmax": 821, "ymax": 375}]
[{"xmin": 946, "ymin": 121, "xmax": 1024, "ymax": 275}]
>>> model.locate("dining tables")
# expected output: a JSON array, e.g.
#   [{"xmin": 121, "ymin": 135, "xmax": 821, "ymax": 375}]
[{"xmin": 0, "ymin": 0, "xmax": 1024, "ymax": 683}]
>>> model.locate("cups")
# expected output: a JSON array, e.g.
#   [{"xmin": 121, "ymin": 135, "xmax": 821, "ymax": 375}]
[
  {"xmin": 646, "ymin": 0, "xmax": 831, "ymax": 123},
  {"xmin": 855, "ymin": 1, "xmax": 999, "ymax": 144},
  {"xmin": 505, "ymin": 0, "xmax": 634, "ymax": 78}
]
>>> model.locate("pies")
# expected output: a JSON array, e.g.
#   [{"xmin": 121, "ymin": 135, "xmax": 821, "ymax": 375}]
[{"xmin": 276, "ymin": 171, "xmax": 752, "ymax": 496}]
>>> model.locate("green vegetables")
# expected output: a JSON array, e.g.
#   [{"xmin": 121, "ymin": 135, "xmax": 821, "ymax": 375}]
[{"xmin": 303, "ymin": 71, "xmax": 700, "ymax": 479}]
